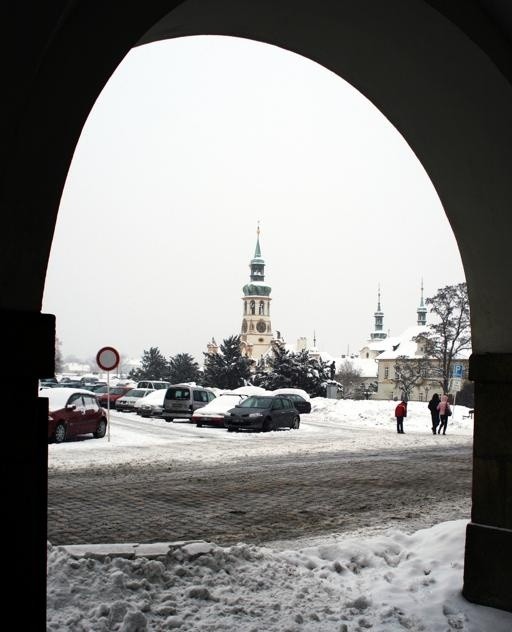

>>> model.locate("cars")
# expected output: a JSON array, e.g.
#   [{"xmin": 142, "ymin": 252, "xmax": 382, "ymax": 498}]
[{"xmin": 39, "ymin": 377, "xmax": 311, "ymax": 444}]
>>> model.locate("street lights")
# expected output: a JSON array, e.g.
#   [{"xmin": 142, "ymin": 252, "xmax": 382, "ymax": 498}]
[{"xmin": 424, "ymin": 386, "xmax": 431, "ymax": 401}]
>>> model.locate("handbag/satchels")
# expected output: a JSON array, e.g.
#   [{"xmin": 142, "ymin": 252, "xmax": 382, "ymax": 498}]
[{"xmin": 445, "ymin": 406, "xmax": 452, "ymax": 417}]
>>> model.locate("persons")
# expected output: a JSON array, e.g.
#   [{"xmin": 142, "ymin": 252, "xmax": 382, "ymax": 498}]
[
  {"xmin": 428, "ymin": 393, "xmax": 453, "ymax": 435},
  {"xmin": 395, "ymin": 400, "xmax": 407, "ymax": 433}
]
